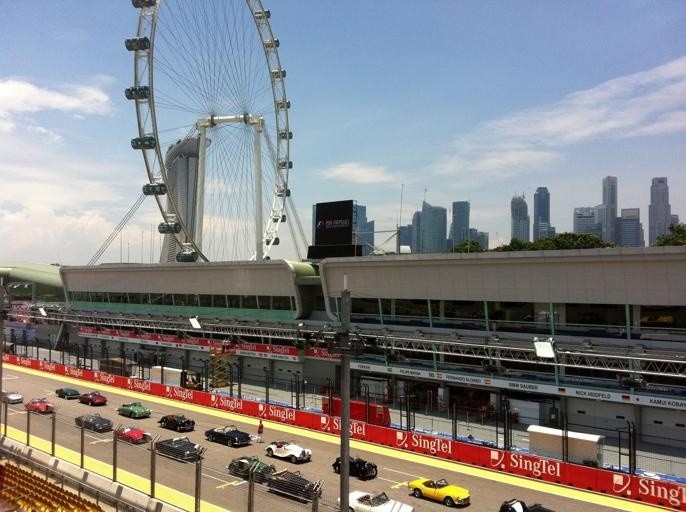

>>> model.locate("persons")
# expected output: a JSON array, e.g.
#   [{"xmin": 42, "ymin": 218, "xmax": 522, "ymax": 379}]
[{"xmin": 257, "ymin": 420, "xmax": 263, "ymax": 443}]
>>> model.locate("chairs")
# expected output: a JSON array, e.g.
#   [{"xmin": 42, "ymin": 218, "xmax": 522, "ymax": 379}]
[{"xmin": 1, "ymin": 462, "xmax": 104, "ymax": 512}]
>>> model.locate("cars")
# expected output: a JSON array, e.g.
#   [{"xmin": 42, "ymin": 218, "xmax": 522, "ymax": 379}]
[
  {"xmin": 336, "ymin": 490, "xmax": 415, "ymax": 512},
  {"xmin": 117, "ymin": 426, "xmax": 152, "ymax": 445},
  {"xmin": 25, "ymin": 398, "xmax": 56, "ymax": 414},
  {"xmin": 229, "ymin": 455, "xmax": 322, "ymax": 504},
  {"xmin": 56, "ymin": 387, "xmax": 108, "ymax": 407},
  {"xmin": 154, "ymin": 436, "xmax": 207, "ymax": 462},
  {"xmin": 157, "ymin": 414, "xmax": 196, "ymax": 432},
  {"xmin": 408, "ymin": 476, "xmax": 471, "ymax": 507},
  {"xmin": 116, "ymin": 401, "xmax": 152, "ymax": 419},
  {"xmin": 333, "ymin": 456, "xmax": 378, "ymax": 480},
  {"xmin": 75, "ymin": 413, "xmax": 113, "ymax": 432},
  {"xmin": 0, "ymin": 387, "xmax": 23, "ymax": 405},
  {"xmin": 205, "ymin": 424, "xmax": 254, "ymax": 448},
  {"xmin": 265, "ymin": 439, "xmax": 313, "ymax": 464}
]
